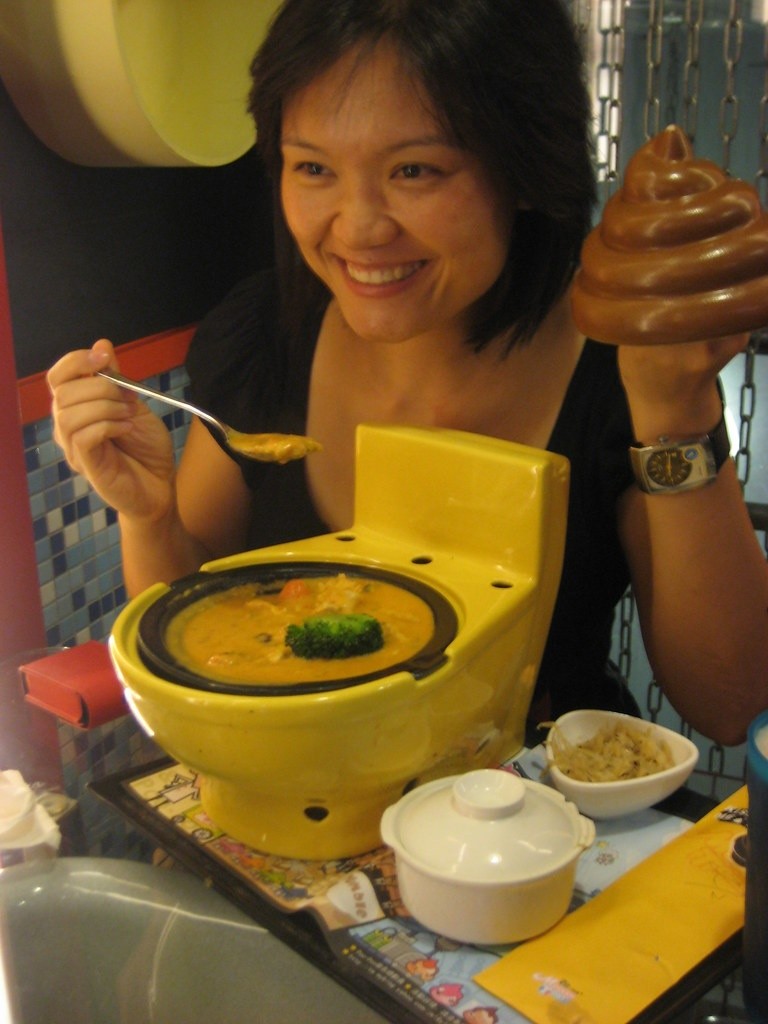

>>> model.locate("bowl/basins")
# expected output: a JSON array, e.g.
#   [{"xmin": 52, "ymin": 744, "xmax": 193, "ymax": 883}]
[{"xmin": 376, "ymin": 767, "xmax": 595, "ymax": 945}]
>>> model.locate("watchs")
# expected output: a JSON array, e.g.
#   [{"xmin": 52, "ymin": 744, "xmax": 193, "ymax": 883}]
[{"xmin": 629, "ymin": 411, "xmax": 731, "ymax": 495}]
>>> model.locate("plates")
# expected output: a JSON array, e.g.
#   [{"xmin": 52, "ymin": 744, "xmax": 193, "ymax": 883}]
[
  {"xmin": 135, "ymin": 561, "xmax": 459, "ymax": 697},
  {"xmin": 546, "ymin": 710, "xmax": 700, "ymax": 820}
]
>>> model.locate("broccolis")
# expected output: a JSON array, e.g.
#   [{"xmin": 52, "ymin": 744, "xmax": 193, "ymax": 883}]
[{"xmin": 282, "ymin": 614, "xmax": 384, "ymax": 659}]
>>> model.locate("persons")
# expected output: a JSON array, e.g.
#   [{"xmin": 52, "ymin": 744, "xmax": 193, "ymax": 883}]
[{"xmin": 47, "ymin": 1, "xmax": 768, "ymax": 753}]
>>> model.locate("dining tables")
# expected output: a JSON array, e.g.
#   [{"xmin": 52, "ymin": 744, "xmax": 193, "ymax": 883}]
[{"xmin": 82, "ymin": 731, "xmax": 751, "ymax": 1024}]
